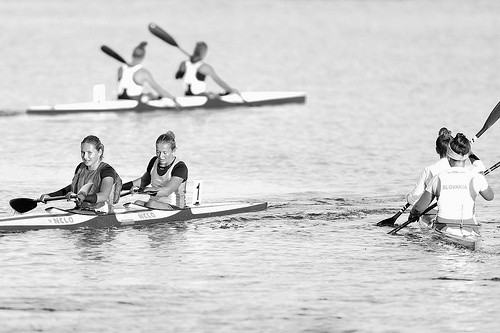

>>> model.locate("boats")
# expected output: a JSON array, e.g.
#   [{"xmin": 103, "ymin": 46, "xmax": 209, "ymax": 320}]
[
  {"xmin": 419, "ymin": 207, "xmax": 480, "ymax": 248},
  {"xmin": 0, "ymin": 199, "xmax": 267, "ymax": 232},
  {"xmin": 27, "ymin": 86, "xmax": 307, "ymax": 115}
]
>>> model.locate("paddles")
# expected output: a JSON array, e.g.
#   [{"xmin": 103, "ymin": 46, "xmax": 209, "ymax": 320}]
[
  {"xmin": 376, "ymin": 101, "xmax": 500, "ymax": 228},
  {"xmin": 387, "ymin": 161, "xmax": 500, "ymax": 235},
  {"xmin": 148, "ymin": 22, "xmax": 248, "ymax": 104},
  {"xmin": 9, "ymin": 195, "xmax": 78, "ymax": 213},
  {"xmin": 120, "ymin": 187, "xmax": 144, "ymax": 197},
  {"xmin": 101, "ymin": 45, "xmax": 182, "ymax": 110}
]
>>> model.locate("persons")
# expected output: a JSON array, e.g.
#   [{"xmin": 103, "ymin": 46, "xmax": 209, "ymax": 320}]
[
  {"xmin": 408, "ymin": 133, "xmax": 494, "ymax": 226},
  {"xmin": 118, "ymin": 41, "xmax": 176, "ymax": 102},
  {"xmin": 120, "ymin": 131, "xmax": 188, "ymax": 211},
  {"xmin": 407, "ymin": 127, "xmax": 486, "ymax": 221},
  {"xmin": 176, "ymin": 42, "xmax": 240, "ymax": 97},
  {"xmin": 37, "ymin": 135, "xmax": 122, "ymax": 216}
]
